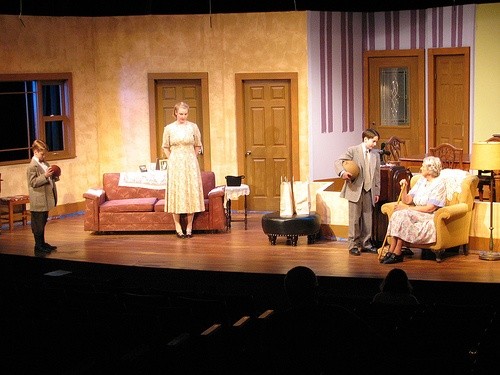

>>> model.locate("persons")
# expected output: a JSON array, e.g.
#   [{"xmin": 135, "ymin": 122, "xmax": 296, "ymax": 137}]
[
  {"xmin": 161, "ymin": 102, "xmax": 206, "ymax": 238},
  {"xmin": 255, "ymin": 267, "xmax": 358, "ymax": 323},
  {"xmin": 381, "ymin": 156, "xmax": 447, "ymax": 264},
  {"xmin": 373, "ymin": 268, "xmax": 429, "ymax": 317},
  {"xmin": 27, "ymin": 140, "xmax": 60, "ymax": 253},
  {"xmin": 333, "ymin": 128, "xmax": 381, "ymax": 256}
]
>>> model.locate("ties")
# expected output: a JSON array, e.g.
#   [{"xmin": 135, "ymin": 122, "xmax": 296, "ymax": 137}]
[{"xmin": 363, "ymin": 151, "xmax": 371, "ymax": 192}]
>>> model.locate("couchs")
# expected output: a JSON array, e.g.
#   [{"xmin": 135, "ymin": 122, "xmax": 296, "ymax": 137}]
[{"xmin": 83, "ymin": 171, "xmax": 225, "ymax": 234}]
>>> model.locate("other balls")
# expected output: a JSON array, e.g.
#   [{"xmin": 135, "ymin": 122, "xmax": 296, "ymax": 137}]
[
  {"xmin": 49, "ymin": 165, "xmax": 61, "ymax": 177},
  {"xmin": 342, "ymin": 161, "xmax": 360, "ymax": 181}
]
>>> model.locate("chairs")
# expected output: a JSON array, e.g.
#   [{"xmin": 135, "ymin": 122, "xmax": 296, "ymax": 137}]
[
  {"xmin": 478, "ymin": 170, "xmax": 495, "ymax": 202},
  {"xmin": 381, "ymin": 168, "xmax": 480, "ymax": 263},
  {"xmin": 429, "ymin": 143, "xmax": 464, "ymax": 170},
  {"xmin": 383, "ymin": 136, "xmax": 408, "ymax": 166}
]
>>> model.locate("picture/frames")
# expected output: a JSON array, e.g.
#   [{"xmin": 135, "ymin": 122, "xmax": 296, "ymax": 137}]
[{"xmin": 159, "ymin": 159, "xmax": 167, "ymax": 171}]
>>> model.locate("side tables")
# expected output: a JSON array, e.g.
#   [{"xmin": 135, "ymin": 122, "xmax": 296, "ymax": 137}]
[{"xmin": 223, "ymin": 183, "xmax": 250, "ymax": 231}]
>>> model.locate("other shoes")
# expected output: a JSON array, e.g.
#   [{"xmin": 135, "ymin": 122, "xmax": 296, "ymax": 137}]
[
  {"xmin": 383, "ymin": 253, "xmax": 403, "ymax": 264},
  {"xmin": 360, "ymin": 247, "xmax": 378, "ymax": 253},
  {"xmin": 176, "ymin": 232, "xmax": 186, "ymax": 239},
  {"xmin": 185, "ymin": 230, "xmax": 192, "ymax": 238},
  {"xmin": 379, "ymin": 252, "xmax": 392, "ymax": 263},
  {"xmin": 348, "ymin": 248, "xmax": 360, "ymax": 256}
]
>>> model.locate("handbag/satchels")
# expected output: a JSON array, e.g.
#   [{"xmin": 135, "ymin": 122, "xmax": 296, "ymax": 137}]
[
  {"xmin": 292, "ymin": 175, "xmax": 311, "ymax": 215},
  {"xmin": 279, "ymin": 175, "xmax": 294, "ymax": 218},
  {"xmin": 225, "ymin": 175, "xmax": 245, "ymax": 186}
]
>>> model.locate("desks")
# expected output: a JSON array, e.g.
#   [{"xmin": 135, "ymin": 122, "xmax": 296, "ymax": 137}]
[
  {"xmin": 0, "ymin": 195, "xmax": 30, "ymax": 232},
  {"xmin": 399, "ymin": 154, "xmax": 470, "ymax": 171}
]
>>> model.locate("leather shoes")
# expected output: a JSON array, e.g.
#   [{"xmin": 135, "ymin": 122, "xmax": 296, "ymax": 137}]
[{"xmin": 34, "ymin": 243, "xmax": 57, "ymax": 254}]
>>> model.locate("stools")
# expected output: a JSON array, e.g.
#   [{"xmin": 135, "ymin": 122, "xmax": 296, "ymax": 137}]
[{"xmin": 262, "ymin": 213, "xmax": 320, "ymax": 246}]
